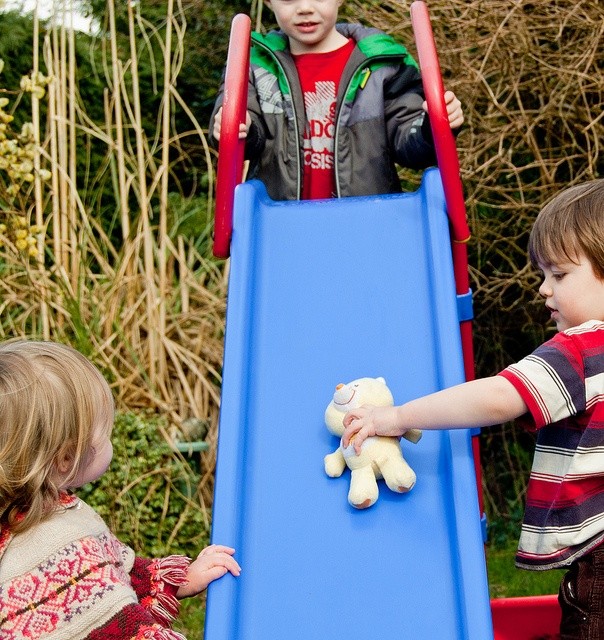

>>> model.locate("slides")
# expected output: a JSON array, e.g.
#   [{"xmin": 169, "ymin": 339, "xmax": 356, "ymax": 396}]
[{"xmin": 203, "ymin": 2, "xmax": 494, "ymax": 639}]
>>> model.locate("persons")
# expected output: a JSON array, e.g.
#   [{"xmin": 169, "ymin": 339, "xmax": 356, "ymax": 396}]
[
  {"xmin": 209, "ymin": 1, "xmax": 464, "ymax": 201},
  {"xmin": 343, "ymin": 179, "xmax": 604, "ymax": 640},
  {"xmin": 0, "ymin": 342, "xmax": 241, "ymax": 640}
]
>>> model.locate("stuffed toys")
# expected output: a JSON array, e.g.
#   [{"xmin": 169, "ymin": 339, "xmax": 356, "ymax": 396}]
[{"xmin": 324, "ymin": 378, "xmax": 423, "ymax": 508}]
